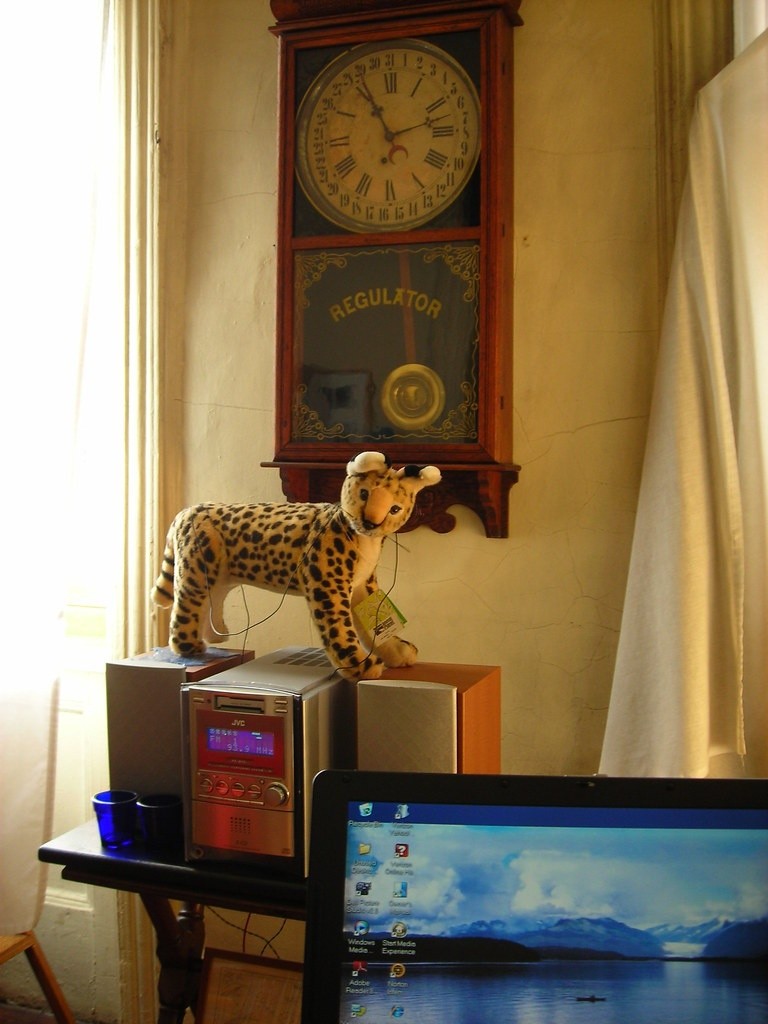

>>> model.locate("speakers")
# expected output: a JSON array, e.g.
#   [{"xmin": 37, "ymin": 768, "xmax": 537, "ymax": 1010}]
[
  {"xmin": 355, "ymin": 663, "xmax": 502, "ymax": 773},
  {"xmin": 104, "ymin": 642, "xmax": 255, "ymax": 828}
]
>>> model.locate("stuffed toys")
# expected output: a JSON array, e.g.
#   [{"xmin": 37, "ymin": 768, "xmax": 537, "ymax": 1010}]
[{"xmin": 150, "ymin": 450, "xmax": 442, "ymax": 683}]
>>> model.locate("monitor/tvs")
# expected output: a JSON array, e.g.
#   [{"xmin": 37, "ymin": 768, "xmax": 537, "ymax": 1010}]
[{"xmin": 302, "ymin": 771, "xmax": 768, "ymax": 1024}]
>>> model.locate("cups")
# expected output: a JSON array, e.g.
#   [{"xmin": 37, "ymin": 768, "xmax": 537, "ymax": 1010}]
[
  {"xmin": 136, "ymin": 792, "xmax": 181, "ymax": 859},
  {"xmin": 92, "ymin": 790, "xmax": 137, "ymax": 850}
]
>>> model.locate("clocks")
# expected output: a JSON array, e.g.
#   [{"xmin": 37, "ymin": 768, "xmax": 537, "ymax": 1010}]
[{"xmin": 263, "ymin": 0, "xmax": 522, "ymax": 546}]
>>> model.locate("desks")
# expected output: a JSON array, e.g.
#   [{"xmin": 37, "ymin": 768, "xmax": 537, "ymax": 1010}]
[{"xmin": 38, "ymin": 819, "xmax": 306, "ymax": 1024}]
